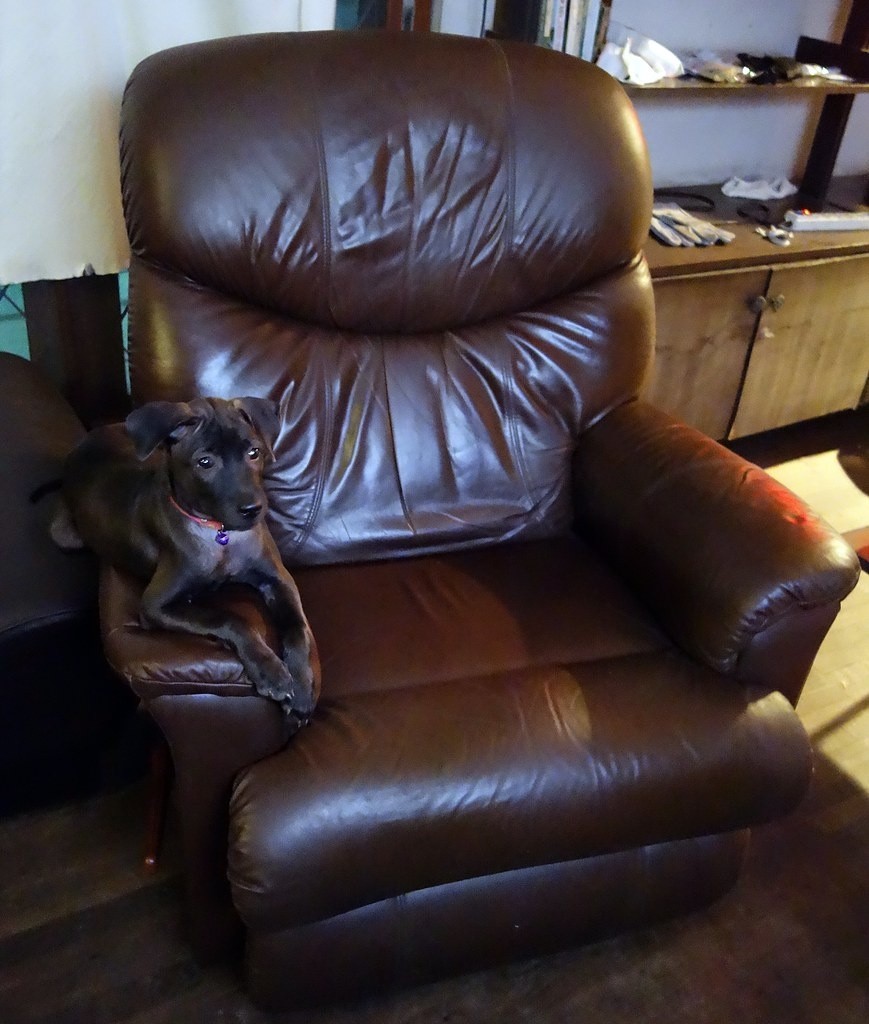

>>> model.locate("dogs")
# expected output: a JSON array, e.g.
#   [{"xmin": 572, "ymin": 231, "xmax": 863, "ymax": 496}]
[{"xmin": 31, "ymin": 394, "xmax": 315, "ymax": 727}]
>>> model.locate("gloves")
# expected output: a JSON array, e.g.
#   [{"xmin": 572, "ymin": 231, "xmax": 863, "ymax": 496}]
[{"xmin": 650, "ymin": 202, "xmax": 736, "ymax": 246}]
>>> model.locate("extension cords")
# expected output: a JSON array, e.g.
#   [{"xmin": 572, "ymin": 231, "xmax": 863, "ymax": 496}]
[{"xmin": 785, "ymin": 208, "xmax": 869, "ymax": 231}]
